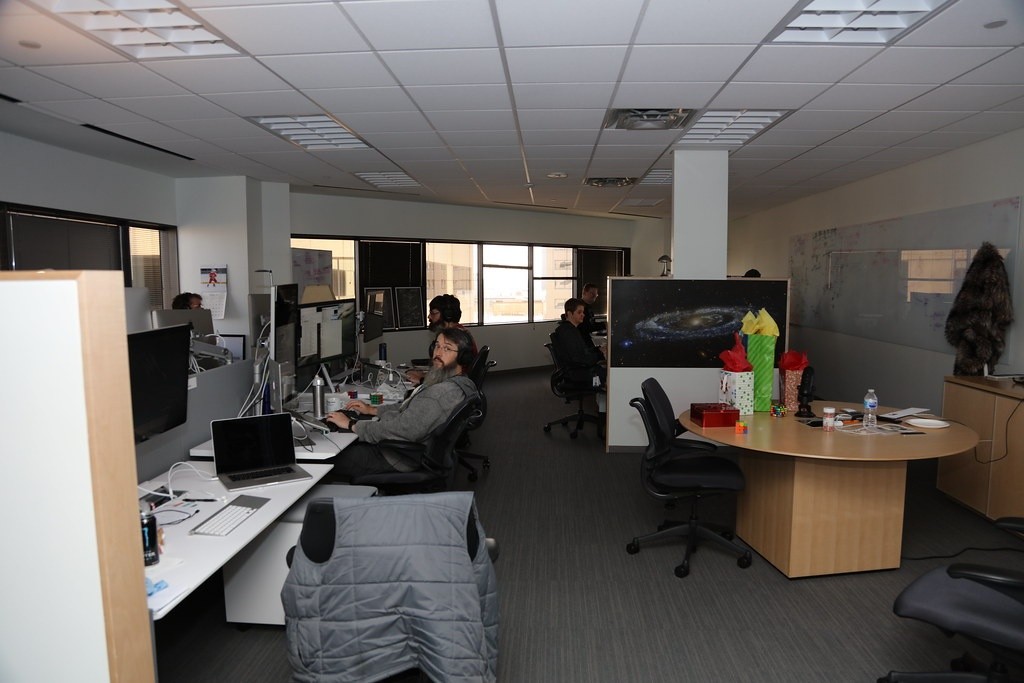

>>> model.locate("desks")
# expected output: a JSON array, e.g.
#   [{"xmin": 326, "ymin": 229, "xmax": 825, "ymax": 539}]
[
  {"xmin": 186, "ymin": 396, "xmax": 398, "ymax": 461},
  {"xmin": 679, "ymin": 400, "xmax": 979, "ymax": 579},
  {"xmin": 296, "ymin": 366, "xmax": 427, "ymax": 401},
  {"xmin": 136, "ymin": 458, "xmax": 334, "ymax": 683}
]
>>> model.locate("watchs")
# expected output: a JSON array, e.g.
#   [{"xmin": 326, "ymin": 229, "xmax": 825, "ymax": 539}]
[{"xmin": 348, "ymin": 420, "xmax": 357, "ymax": 433}]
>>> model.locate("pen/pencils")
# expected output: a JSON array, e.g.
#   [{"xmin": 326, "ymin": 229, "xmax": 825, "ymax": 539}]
[{"xmin": 182, "ymin": 499, "xmax": 217, "ymax": 502}]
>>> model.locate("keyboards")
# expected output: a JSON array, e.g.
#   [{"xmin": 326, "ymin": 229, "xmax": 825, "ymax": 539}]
[
  {"xmin": 188, "ymin": 495, "xmax": 271, "ymax": 536},
  {"xmin": 315, "ymin": 409, "xmax": 359, "ymax": 432}
]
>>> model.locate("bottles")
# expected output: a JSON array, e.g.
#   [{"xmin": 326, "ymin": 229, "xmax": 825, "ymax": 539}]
[
  {"xmin": 863, "ymin": 389, "xmax": 879, "ymax": 430},
  {"xmin": 379, "ymin": 343, "xmax": 386, "ymax": 360},
  {"xmin": 823, "ymin": 407, "xmax": 836, "ymax": 432}
]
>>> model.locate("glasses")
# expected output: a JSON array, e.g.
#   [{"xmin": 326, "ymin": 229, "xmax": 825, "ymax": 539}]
[
  {"xmin": 585, "ymin": 292, "xmax": 598, "ymax": 299},
  {"xmin": 431, "ymin": 342, "xmax": 458, "ymax": 354},
  {"xmin": 430, "ymin": 310, "xmax": 440, "ymax": 318},
  {"xmin": 190, "ymin": 304, "xmax": 204, "ymax": 309}
]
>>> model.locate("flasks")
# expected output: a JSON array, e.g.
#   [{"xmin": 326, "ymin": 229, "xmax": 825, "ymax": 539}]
[{"xmin": 313, "ymin": 375, "xmax": 326, "ymax": 418}]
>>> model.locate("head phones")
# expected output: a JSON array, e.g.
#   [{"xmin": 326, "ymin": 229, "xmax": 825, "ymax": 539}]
[
  {"xmin": 429, "ymin": 327, "xmax": 473, "ymax": 365},
  {"xmin": 440, "ymin": 294, "xmax": 457, "ymax": 322}
]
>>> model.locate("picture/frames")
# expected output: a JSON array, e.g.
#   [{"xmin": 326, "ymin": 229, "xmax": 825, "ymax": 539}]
[
  {"xmin": 394, "ymin": 286, "xmax": 426, "ymax": 331},
  {"xmin": 364, "ymin": 286, "xmax": 395, "ymax": 332}
]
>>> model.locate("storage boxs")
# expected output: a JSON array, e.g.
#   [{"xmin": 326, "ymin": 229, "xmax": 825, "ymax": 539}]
[{"xmin": 690, "ymin": 401, "xmax": 740, "ymax": 428}]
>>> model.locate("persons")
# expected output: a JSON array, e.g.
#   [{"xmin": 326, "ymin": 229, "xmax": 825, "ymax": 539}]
[
  {"xmin": 578, "ymin": 283, "xmax": 606, "ymax": 345},
  {"xmin": 326, "ymin": 325, "xmax": 479, "ymax": 478},
  {"xmin": 553, "ymin": 298, "xmax": 607, "ymax": 384},
  {"xmin": 406, "ymin": 294, "xmax": 478, "ymax": 384},
  {"xmin": 171, "ymin": 292, "xmax": 203, "ymax": 309}
]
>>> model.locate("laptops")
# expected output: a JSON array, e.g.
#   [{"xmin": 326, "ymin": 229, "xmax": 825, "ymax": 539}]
[{"xmin": 211, "ymin": 412, "xmax": 313, "ymax": 492}]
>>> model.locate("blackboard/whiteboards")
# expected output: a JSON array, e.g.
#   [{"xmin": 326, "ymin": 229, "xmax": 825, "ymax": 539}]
[
  {"xmin": 786, "ymin": 194, "xmax": 1021, "ymax": 365},
  {"xmin": 394, "ymin": 287, "xmax": 426, "ymax": 329},
  {"xmin": 364, "ymin": 287, "xmax": 395, "ymax": 331}
]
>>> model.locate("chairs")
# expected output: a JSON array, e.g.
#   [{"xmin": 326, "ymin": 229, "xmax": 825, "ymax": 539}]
[
  {"xmin": 625, "ymin": 397, "xmax": 752, "ymax": 578},
  {"xmin": 351, "ymin": 393, "xmax": 479, "ymax": 497},
  {"xmin": 542, "ymin": 330, "xmax": 604, "ymax": 440},
  {"xmin": 469, "ymin": 345, "xmax": 496, "ymax": 389},
  {"xmin": 284, "ymin": 500, "xmax": 498, "ymax": 683},
  {"xmin": 639, "ymin": 376, "xmax": 718, "ymax": 510},
  {"xmin": 874, "ymin": 516, "xmax": 1024, "ymax": 683}
]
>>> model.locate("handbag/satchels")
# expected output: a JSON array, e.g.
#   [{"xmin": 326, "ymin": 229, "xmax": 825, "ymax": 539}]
[{"xmin": 719, "ymin": 331, "xmax": 808, "ymax": 416}]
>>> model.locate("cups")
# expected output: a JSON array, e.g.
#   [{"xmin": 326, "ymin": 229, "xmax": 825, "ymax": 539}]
[{"xmin": 327, "ymin": 398, "xmax": 345, "ymax": 412}]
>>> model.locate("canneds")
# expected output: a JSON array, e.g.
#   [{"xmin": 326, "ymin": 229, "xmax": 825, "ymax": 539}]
[
  {"xmin": 822, "ymin": 406, "xmax": 835, "ymax": 432},
  {"xmin": 141, "ymin": 512, "xmax": 160, "ymax": 568}
]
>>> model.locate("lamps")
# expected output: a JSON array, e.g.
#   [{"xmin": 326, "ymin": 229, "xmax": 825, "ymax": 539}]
[{"xmin": 300, "ymin": 284, "xmax": 337, "ymax": 372}]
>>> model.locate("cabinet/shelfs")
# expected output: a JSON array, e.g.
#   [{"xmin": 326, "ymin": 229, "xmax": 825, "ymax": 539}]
[{"xmin": 934, "ymin": 376, "xmax": 1024, "ymax": 537}]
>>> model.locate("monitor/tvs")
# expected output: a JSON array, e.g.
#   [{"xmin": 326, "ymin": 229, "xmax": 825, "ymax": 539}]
[
  {"xmin": 363, "ymin": 290, "xmax": 385, "ymax": 342},
  {"xmin": 248, "ymin": 293, "xmax": 271, "ymax": 347},
  {"xmin": 127, "ymin": 324, "xmax": 190, "ymax": 445},
  {"xmin": 272, "ymin": 283, "xmax": 299, "ymax": 364},
  {"xmin": 151, "ymin": 308, "xmax": 217, "ymax": 357},
  {"xmin": 296, "ymin": 298, "xmax": 359, "ymax": 368}
]
